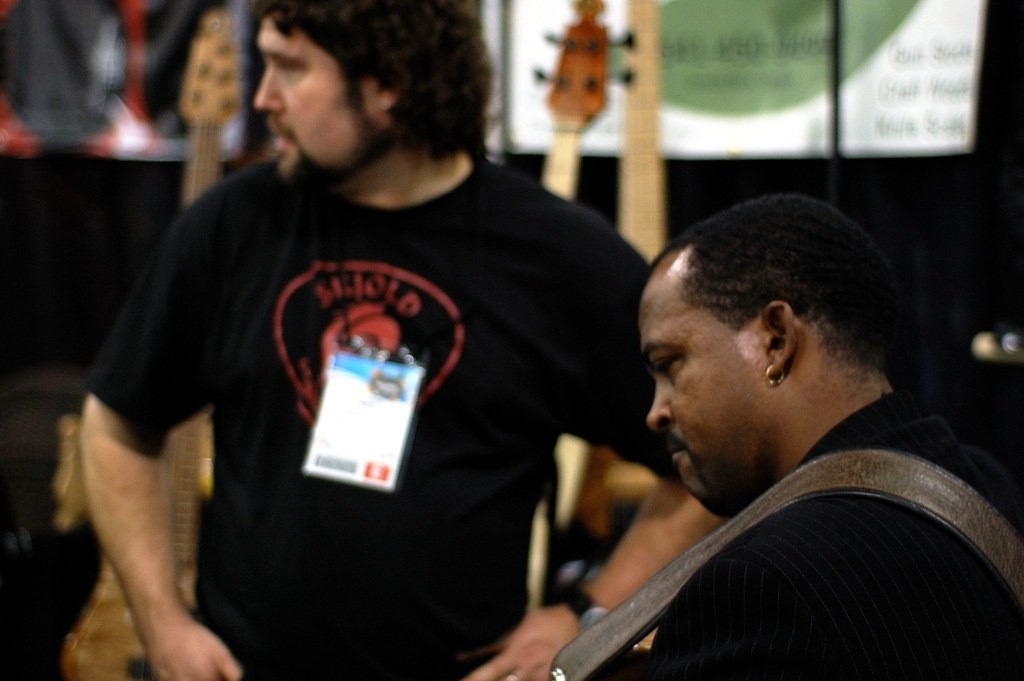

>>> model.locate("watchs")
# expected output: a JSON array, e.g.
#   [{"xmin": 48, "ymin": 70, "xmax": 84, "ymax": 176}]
[{"xmin": 568, "ymin": 590, "xmax": 609, "ymax": 627}]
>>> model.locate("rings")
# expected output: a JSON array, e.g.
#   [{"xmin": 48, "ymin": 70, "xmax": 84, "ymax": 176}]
[{"xmin": 505, "ymin": 672, "xmax": 518, "ymax": 681}]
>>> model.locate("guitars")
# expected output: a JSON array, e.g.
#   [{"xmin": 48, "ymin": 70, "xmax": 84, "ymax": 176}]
[
  {"xmin": 58, "ymin": 7, "xmax": 241, "ymax": 680},
  {"xmin": 533, "ymin": 1, "xmax": 638, "ymax": 540}
]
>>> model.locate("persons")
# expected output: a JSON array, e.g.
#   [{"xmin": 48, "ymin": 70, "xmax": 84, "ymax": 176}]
[
  {"xmin": 79, "ymin": 0, "xmax": 725, "ymax": 681},
  {"xmin": 640, "ymin": 192, "xmax": 1023, "ymax": 681}
]
